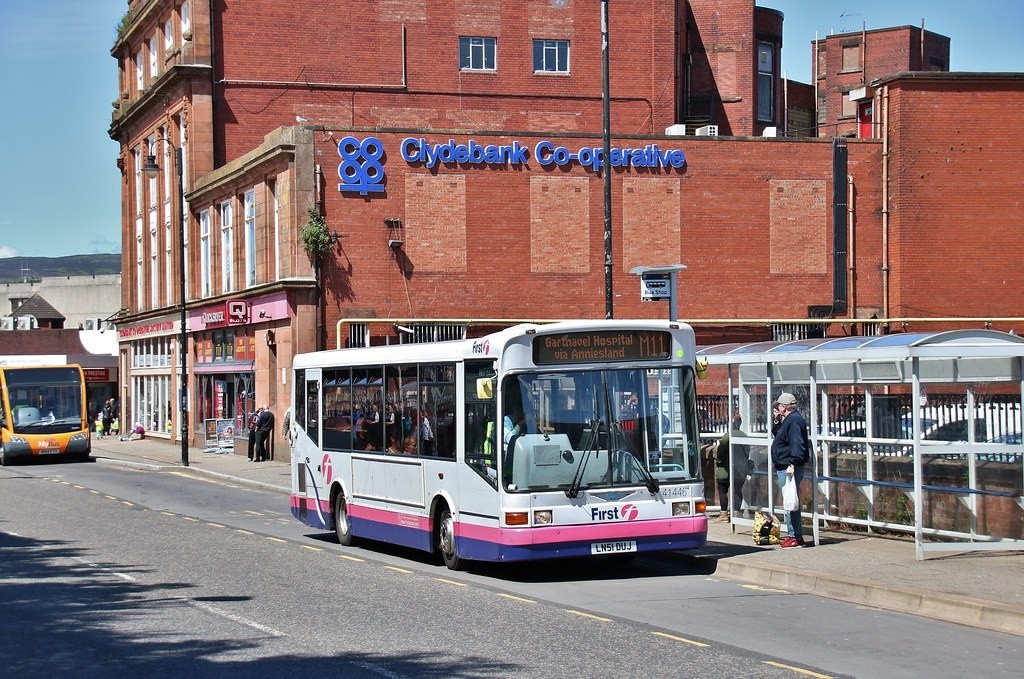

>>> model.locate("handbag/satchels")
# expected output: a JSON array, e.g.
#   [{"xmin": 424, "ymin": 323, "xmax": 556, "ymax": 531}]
[
  {"xmin": 781, "ymin": 474, "xmax": 799, "ymax": 512},
  {"xmin": 754, "ymin": 510, "xmax": 782, "ymax": 544},
  {"xmin": 747, "ymin": 459, "xmax": 754, "ymax": 475},
  {"xmin": 112, "ymin": 418, "xmax": 119, "ymax": 430}
]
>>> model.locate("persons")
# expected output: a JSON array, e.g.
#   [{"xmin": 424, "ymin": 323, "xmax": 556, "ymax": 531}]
[
  {"xmin": 246, "ymin": 405, "xmax": 274, "ymax": 462},
  {"xmin": 362, "ymin": 435, "xmax": 382, "ymax": 451},
  {"xmin": 121, "ymin": 422, "xmax": 145, "ymax": 441},
  {"xmin": 631, "ymin": 392, "xmax": 657, "ymax": 451},
  {"xmin": 353, "ymin": 402, "xmax": 435, "ymax": 456},
  {"xmin": 503, "ymin": 402, "xmax": 540, "ymax": 460},
  {"xmin": 771, "ymin": 393, "xmax": 809, "ymax": 550},
  {"xmin": 402, "ymin": 435, "xmax": 417, "ymax": 455},
  {"xmin": 94, "ymin": 398, "xmax": 119, "ymax": 440},
  {"xmin": 712, "ymin": 412, "xmax": 750, "ymax": 522},
  {"xmin": 386, "ymin": 426, "xmax": 402, "ymax": 454},
  {"xmin": 281, "ymin": 405, "xmax": 291, "ymax": 447}
]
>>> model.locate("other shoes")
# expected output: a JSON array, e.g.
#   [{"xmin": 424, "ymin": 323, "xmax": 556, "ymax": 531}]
[
  {"xmin": 254, "ymin": 459, "xmax": 260, "ymax": 462},
  {"xmin": 261, "ymin": 457, "xmax": 266, "ymax": 461},
  {"xmin": 247, "ymin": 458, "xmax": 252, "ymax": 462},
  {"xmin": 97, "ymin": 437, "xmax": 100, "ymax": 440}
]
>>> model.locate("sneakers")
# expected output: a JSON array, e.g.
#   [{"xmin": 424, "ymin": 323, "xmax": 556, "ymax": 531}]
[{"xmin": 780, "ymin": 537, "xmax": 806, "ymax": 549}]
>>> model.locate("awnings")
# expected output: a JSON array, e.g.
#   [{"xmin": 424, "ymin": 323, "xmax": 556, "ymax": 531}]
[{"xmin": 849, "ymin": 86, "xmax": 875, "ymax": 102}]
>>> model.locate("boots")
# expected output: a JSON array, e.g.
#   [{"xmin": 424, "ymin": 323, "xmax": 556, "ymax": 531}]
[
  {"xmin": 715, "ymin": 510, "xmax": 729, "ymax": 522},
  {"xmin": 734, "ymin": 510, "xmax": 741, "ymax": 518}
]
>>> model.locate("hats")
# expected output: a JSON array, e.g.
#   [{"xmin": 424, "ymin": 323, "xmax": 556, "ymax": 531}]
[{"xmin": 772, "ymin": 393, "xmax": 797, "ymax": 405}]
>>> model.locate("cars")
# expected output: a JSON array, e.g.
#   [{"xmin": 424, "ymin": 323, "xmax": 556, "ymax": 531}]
[{"xmin": 776, "ymin": 403, "xmax": 1024, "ymax": 463}]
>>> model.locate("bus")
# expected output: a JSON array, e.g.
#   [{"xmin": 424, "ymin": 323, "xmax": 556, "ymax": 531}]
[
  {"xmin": 0, "ymin": 363, "xmax": 92, "ymax": 465},
  {"xmin": 290, "ymin": 321, "xmax": 711, "ymax": 572}
]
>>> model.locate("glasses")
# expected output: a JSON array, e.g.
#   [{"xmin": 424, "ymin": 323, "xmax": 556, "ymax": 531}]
[{"xmin": 259, "ymin": 408, "xmax": 264, "ymax": 410}]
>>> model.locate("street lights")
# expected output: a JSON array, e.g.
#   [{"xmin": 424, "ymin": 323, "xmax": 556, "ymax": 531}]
[{"xmin": 141, "ymin": 138, "xmax": 189, "ymax": 468}]
